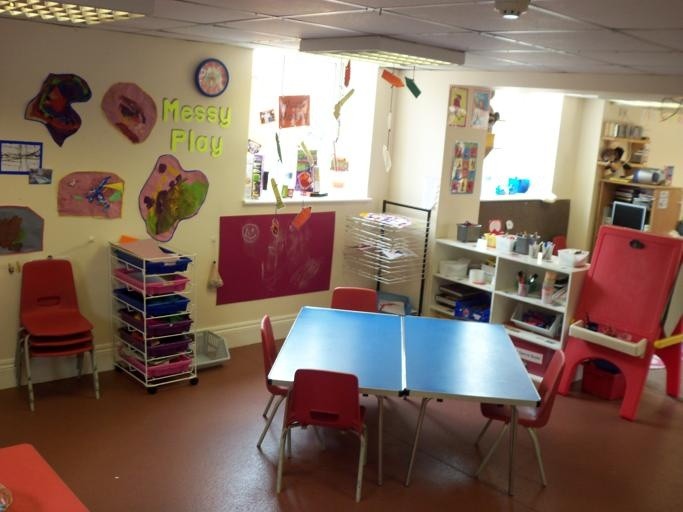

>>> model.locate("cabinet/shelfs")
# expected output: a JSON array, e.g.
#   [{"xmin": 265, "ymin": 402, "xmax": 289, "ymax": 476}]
[
  {"xmin": 588, "ymin": 174, "xmax": 683, "ymax": 264},
  {"xmin": 594, "ymin": 132, "xmax": 651, "ymax": 172},
  {"xmin": 427, "ymin": 232, "xmax": 592, "ymax": 390},
  {"xmin": 105, "ymin": 242, "xmax": 199, "ymax": 396}
]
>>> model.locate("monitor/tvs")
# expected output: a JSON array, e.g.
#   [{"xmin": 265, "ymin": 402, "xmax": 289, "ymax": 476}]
[{"xmin": 611, "ymin": 201, "xmax": 646, "ymax": 230}]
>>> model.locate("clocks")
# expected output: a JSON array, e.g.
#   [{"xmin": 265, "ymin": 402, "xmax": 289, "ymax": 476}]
[{"xmin": 194, "ymin": 57, "xmax": 229, "ymax": 98}]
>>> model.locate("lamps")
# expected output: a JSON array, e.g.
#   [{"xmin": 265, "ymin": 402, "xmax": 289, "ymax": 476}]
[
  {"xmin": 0, "ymin": 1, "xmax": 156, "ymax": 31},
  {"xmin": 296, "ymin": 34, "xmax": 465, "ymax": 71},
  {"xmin": 596, "ymin": 91, "xmax": 682, "ymax": 110}
]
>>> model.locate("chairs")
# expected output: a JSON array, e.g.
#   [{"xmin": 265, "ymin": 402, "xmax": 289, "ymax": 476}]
[
  {"xmin": 12, "ymin": 256, "xmax": 102, "ymax": 414},
  {"xmin": 465, "ymin": 347, "xmax": 569, "ymax": 489},
  {"xmin": 329, "ymin": 286, "xmax": 389, "ymax": 402},
  {"xmin": 253, "ymin": 313, "xmax": 294, "ymax": 448},
  {"xmin": 275, "ymin": 366, "xmax": 371, "ymax": 505}
]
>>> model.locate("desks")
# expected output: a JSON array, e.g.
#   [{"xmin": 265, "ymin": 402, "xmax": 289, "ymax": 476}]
[
  {"xmin": 0, "ymin": 441, "xmax": 94, "ymax": 512},
  {"xmin": 396, "ymin": 312, "xmax": 549, "ymax": 490},
  {"xmin": 263, "ymin": 304, "xmax": 404, "ymax": 487}
]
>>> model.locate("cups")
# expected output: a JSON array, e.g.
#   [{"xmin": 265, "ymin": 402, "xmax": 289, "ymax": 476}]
[
  {"xmin": 529, "ymin": 243, "xmax": 539, "ymax": 258},
  {"xmin": 541, "ymin": 271, "xmax": 556, "ymax": 303},
  {"xmin": 517, "ymin": 283, "xmax": 529, "ymax": 297},
  {"xmin": 542, "ymin": 246, "xmax": 554, "ymax": 261},
  {"xmin": 469, "ymin": 269, "xmax": 485, "ymax": 284}
]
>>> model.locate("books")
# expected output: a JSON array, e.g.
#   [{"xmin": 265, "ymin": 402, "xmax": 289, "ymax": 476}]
[
  {"xmin": 435, "ymin": 284, "xmax": 487, "ymax": 313},
  {"xmin": 610, "ymin": 184, "xmax": 654, "ymax": 212},
  {"xmin": 603, "ymin": 119, "xmax": 644, "ymax": 140}
]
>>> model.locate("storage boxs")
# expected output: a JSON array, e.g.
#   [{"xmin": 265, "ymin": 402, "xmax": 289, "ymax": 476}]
[{"xmin": 580, "ymin": 359, "xmax": 626, "ymax": 401}]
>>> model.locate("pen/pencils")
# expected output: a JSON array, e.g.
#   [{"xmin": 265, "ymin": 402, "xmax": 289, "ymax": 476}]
[
  {"xmin": 516, "ymin": 270, "xmax": 538, "ymax": 285},
  {"xmin": 520, "ymin": 230, "xmax": 538, "ymax": 239},
  {"xmin": 532, "ymin": 241, "xmax": 556, "ymax": 260}
]
